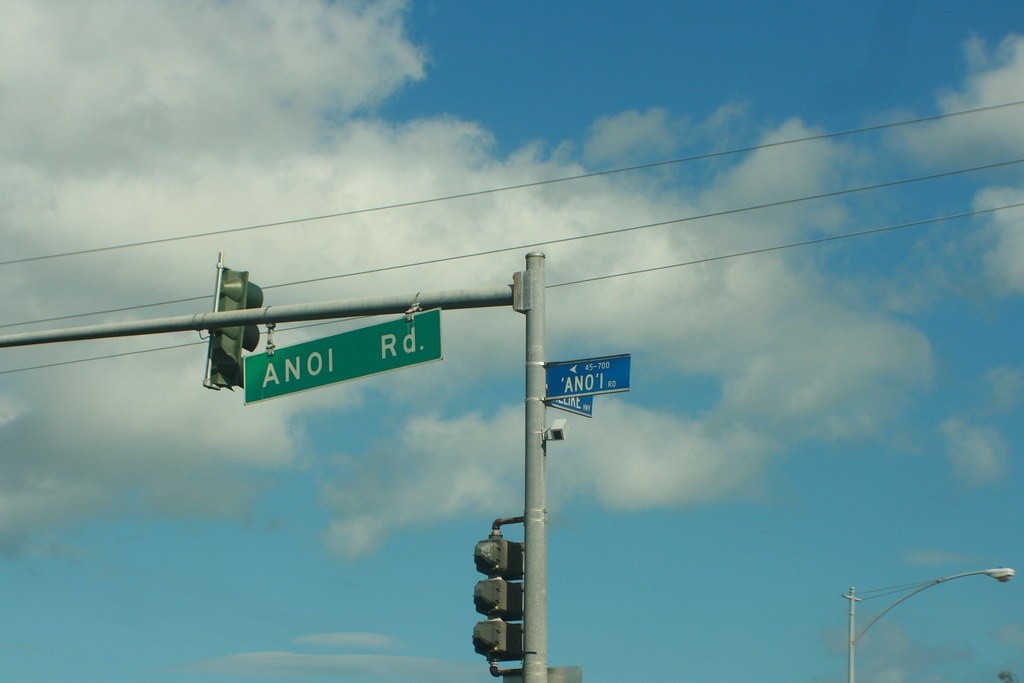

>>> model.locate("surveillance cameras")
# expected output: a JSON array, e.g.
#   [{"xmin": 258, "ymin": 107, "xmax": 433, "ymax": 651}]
[{"xmin": 549, "ymin": 418, "xmax": 570, "ymax": 442}]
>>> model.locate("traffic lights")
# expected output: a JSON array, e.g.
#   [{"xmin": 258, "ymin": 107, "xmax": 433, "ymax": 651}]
[{"xmin": 204, "ymin": 264, "xmax": 265, "ymax": 394}]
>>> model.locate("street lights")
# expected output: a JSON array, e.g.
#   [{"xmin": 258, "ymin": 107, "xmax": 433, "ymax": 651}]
[{"xmin": 842, "ymin": 567, "xmax": 1015, "ymax": 683}]
